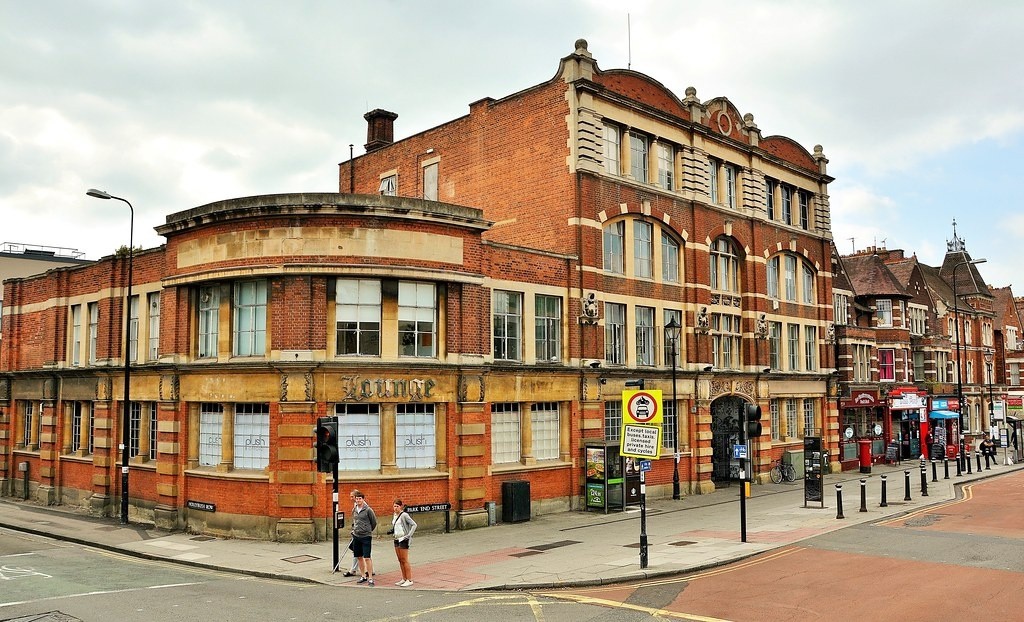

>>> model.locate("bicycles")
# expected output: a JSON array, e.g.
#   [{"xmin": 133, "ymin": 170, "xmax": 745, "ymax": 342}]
[{"xmin": 770, "ymin": 459, "xmax": 796, "ymax": 484}]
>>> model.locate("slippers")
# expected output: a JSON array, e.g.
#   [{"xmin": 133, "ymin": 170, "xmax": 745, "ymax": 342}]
[
  {"xmin": 365, "ymin": 571, "xmax": 375, "ymax": 577},
  {"xmin": 343, "ymin": 570, "xmax": 357, "ymax": 577}
]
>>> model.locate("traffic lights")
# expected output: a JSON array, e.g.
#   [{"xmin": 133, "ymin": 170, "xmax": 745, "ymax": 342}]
[
  {"xmin": 745, "ymin": 403, "xmax": 762, "ymax": 439},
  {"xmin": 313, "ymin": 416, "xmax": 338, "ymax": 473}
]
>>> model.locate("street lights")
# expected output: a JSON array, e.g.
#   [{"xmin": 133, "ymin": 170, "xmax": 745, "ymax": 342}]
[
  {"xmin": 983, "ymin": 348, "xmax": 997, "ymax": 454},
  {"xmin": 625, "ymin": 379, "xmax": 649, "ymax": 569},
  {"xmin": 953, "ymin": 258, "xmax": 987, "ymax": 471},
  {"xmin": 665, "ymin": 315, "xmax": 683, "ymax": 500},
  {"xmin": 86, "ymin": 189, "xmax": 134, "ymax": 524}
]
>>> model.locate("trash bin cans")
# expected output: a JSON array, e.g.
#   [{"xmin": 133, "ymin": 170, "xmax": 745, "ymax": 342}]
[{"xmin": 502, "ymin": 480, "xmax": 530, "ymax": 523}]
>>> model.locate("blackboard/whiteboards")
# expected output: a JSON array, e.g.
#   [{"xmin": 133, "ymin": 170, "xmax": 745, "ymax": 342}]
[
  {"xmin": 931, "ymin": 443, "xmax": 945, "ymax": 460},
  {"xmin": 885, "ymin": 443, "xmax": 899, "ymax": 461}
]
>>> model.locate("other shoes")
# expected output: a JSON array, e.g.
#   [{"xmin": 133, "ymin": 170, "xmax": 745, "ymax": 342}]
[
  {"xmin": 400, "ymin": 580, "xmax": 414, "ymax": 587},
  {"xmin": 356, "ymin": 576, "xmax": 368, "ymax": 584},
  {"xmin": 395, "ymin": 579, "xmax": 406, "ymax": 586},
  {"xmin": 367, "ymin": 578, "xmax": 375, "ymax": 585}
]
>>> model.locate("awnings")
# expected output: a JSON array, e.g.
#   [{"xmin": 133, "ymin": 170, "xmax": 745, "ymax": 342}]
[{"xmin": 929, "ymin": 411, "xmax": 959, "ymax": 419}]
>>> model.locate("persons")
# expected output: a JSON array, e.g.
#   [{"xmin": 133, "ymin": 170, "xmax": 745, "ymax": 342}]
[
  {"xmin": 342, "ymin": 488, "xmax": 376, "ymax": 587},
  {"xmin": 392, "ymin": 499, "xmax": 418, "ymax": 587},
  {"xmin": 983, "ymin": 428, "xmax": 1016, "ymax": 465}
]
